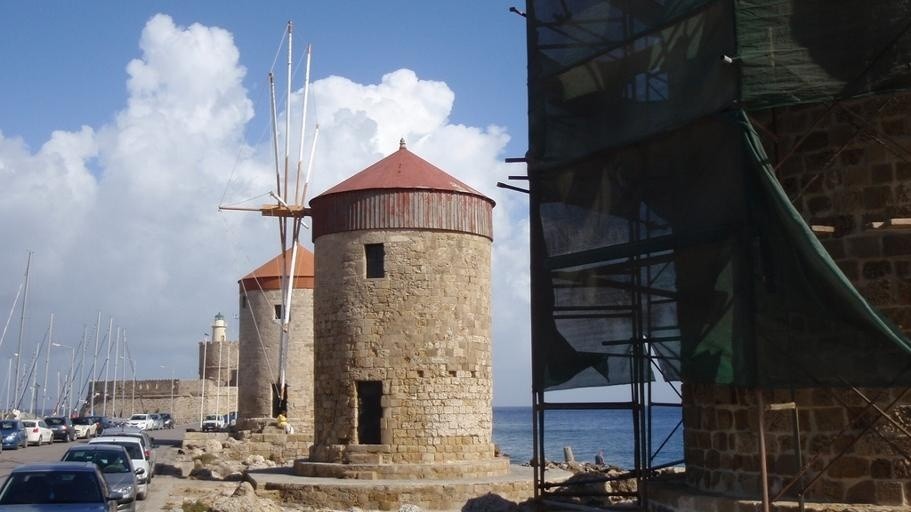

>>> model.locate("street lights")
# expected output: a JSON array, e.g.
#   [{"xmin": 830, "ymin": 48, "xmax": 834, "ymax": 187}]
[
  {"xmin": 51, "ymin": 342, "xmax": 74, "ymax": 418},
  {"xmin": 159, "ymin": 361, "xmax": 175, "ymax": 421},
  {"xmin": 199, "ymin": 328, "xmax": 240, "ymax": 431},
  {"xmin": 118, "ymin": 355, "xmax": 135, "ymax": 416}
]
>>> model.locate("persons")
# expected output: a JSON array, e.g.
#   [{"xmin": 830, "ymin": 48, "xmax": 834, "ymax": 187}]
[
  {"xmin": 595, "ymin": 450, "xmax": 606, "ymax": 467},
  {"xmin": 107, "ymin": 421, "xmax": 112, "ymax": 428},
  {"xmin": 95, "ymin": 421, "xmax": 102, "ymax": 434},
  {"xmin": 278, "ymin": 410, "xmax": 295, "ymax": 434}
]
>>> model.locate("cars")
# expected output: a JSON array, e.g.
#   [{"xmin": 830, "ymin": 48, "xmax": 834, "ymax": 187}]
[
  {"xmin": 89, "ymin": 436, "xmax": 150, "ymax": 501},
  {"xmin": 57, "ymin": 443, "xmax": 136, "ymax": 512},
  {"xmin": 0, "ymin": 409, "xmax": 175, "ymax": 449},
  {"xmin": 1, "ymin": 461, "xmax": 122, "ymax": 511},
  {"xmin": 101, "ymin": 426, "xmax": 155, "ymax": 476}
]
